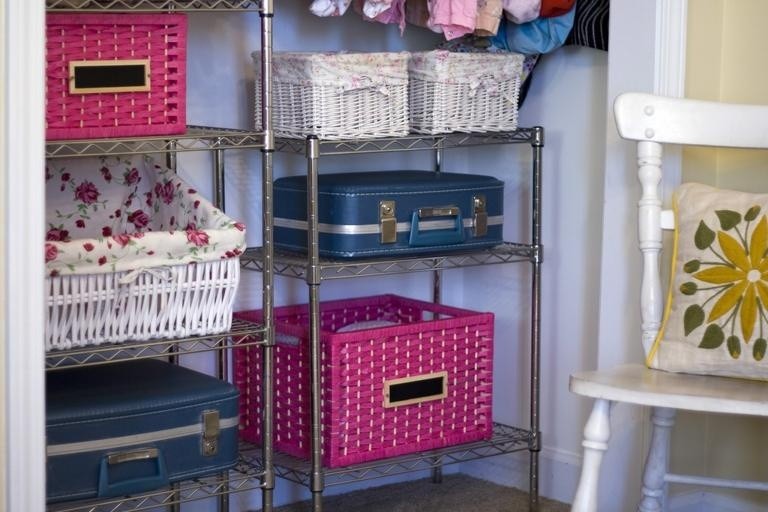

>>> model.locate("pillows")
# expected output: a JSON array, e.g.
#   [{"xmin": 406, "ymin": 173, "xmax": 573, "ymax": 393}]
[{"xmin": 646, "ymin": 180, "xmax": 767, "ymax": 381}]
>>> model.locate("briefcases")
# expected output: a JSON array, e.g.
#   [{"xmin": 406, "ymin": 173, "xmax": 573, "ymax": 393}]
[
  {"xmin": 273, "ymin": 169, "xmax": 504, "ymax": 258},
  {"xmin": 46, "ymin": 359, "xmax": 235, "ymax": 505}
]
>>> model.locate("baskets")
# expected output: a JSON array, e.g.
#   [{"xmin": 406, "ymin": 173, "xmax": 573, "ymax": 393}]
[
  {"xmin": 410, "ymin": 51, "xmax": 525, "ymax": 134},
  {"xmin": 251, "ymin": 50, "xmax": 409, "ymax": 138},
  {"xmin": 44, "ymin": 154, "xmax": 246, "ymax": 352}
]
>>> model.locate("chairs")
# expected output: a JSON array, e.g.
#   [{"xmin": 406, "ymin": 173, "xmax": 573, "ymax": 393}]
[{"xmin": 566, "ymin": 90, "xmax": 768, "ymax": 512}]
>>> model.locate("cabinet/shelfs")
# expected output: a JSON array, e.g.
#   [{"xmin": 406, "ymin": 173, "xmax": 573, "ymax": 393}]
[
  {"xmin": 47, "ymin": 0, "xmax": 276, "ymax": 512},
  {"xmin": 213, "ymin": 127, "xmax": 545, "ymax": 512}
]
